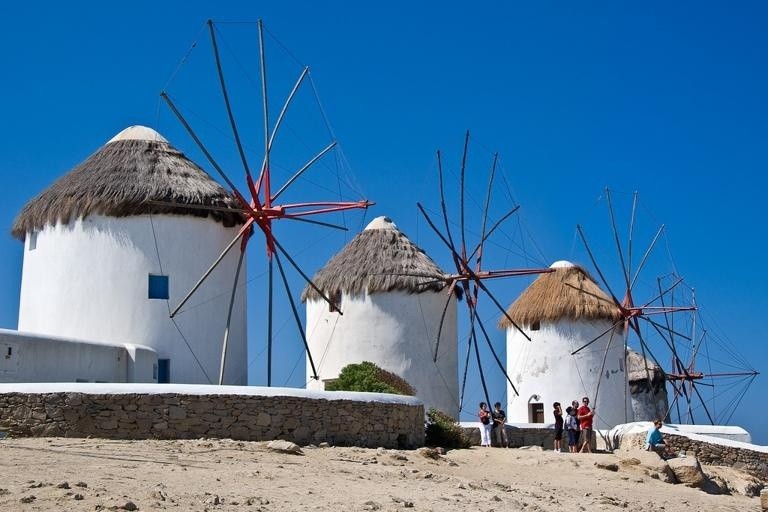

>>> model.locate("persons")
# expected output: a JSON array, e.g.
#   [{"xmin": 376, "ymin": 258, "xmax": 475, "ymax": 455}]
[
  {"xmin": 477, "ymin": 401, "xmax": 493, "ymax": 448},
  {"xmin": 572, "ymin": 400, "xmax": 582, "ymax": 452},
  {"xmin": 491, "ymin": 402, "xmax": 509, "ymax": 448},
  {"xmin": 553, "ymin": 401, "xmax": 564, "ymax": 453},
  {"xmin": 645, "ymin": 418, "xmax": 678, "ymax": 460},
  {"xmin": 565, "ymin": 407, "xmax": 578, "ymax": 453},
  {"xmin": 576, "ymin": 396, "xmax": 596, "ymax": 453}
]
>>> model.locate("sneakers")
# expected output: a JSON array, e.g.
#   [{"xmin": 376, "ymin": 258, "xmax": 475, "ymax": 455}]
[{"xmin": 554, "ymin": 448, "xmax": 562, "ymax": 453}]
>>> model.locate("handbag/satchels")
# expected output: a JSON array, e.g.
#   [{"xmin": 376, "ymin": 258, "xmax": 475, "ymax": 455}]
[
  {"xmin": 482, "ymin": 416, "xmax": 490, "ymax": 425},
  {"xmin": 493, "ymin": 422, "xmax": 499, "ymax": 429}
]
